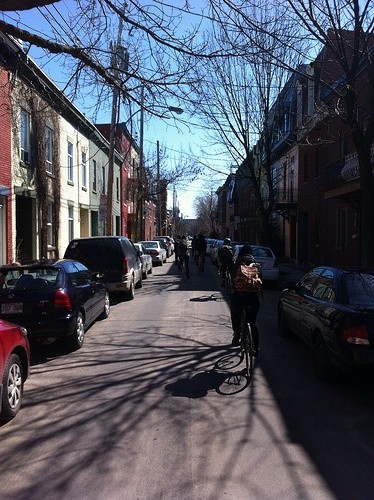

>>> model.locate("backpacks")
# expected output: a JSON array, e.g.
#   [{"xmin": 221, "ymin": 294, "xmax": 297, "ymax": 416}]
[{"xmin": 233, "ymin": 261, "xmax": 262, "ymax": 292}]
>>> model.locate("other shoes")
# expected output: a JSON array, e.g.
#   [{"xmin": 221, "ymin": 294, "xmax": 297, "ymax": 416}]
[{"xmin": 232, "ymin": 336, "xmax": 239, "ymax": 343}]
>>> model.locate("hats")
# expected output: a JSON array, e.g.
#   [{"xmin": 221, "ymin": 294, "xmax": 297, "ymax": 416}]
[
  {"xmin": 224, "ymin": 238, "xmax": 231, "ymax": 245},
  {"xmin": 239, "ymin": 245, "xmax": 252, "ymax": 254}
]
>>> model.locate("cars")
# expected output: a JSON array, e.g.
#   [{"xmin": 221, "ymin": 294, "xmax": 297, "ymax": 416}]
[
  {"xmin": 205, "ymin": 238, "xmax": 217, "ymax": 254},
  {"xmin": 63, "ymin": 235, "xmax": 144, "ymax": 301},
  {"xmin": 277, "ymin": 263, "xmax": 374, "ymax": 382},
  {"xmin": 132, "ymin": 243, "xmax": 154, "ymax": 279},
  {"xmin": 137, "ymin": 240, "xmax": 167, "ymax": 266},
  {"xmin": 153, "ymin": 238, "xmax": 172, "ymax": 258},
  {"xmin": 232, "ymin": 244, "xmax": 279, "ymax": 287},
  {"xmin": 159, "ymin": 235, "xmax": 175, "ymax": 252},
  {"xmin": 0, "ymin": 258, "xmax": 111, "ymax": 351},
  {"xmin": 210, "ymin": 240, "xmax": 239, "ymax": 263},
  {"xmin": 0, "ymin": 315, "xmax": 33, "ymax": 422}
]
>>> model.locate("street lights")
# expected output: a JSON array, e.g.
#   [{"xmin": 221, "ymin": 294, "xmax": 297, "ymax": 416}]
[{"xmin": 105, "ymin": 104, "xmax": 181, "ymax": 236}]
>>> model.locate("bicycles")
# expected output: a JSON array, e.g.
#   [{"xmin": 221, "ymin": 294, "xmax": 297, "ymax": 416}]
[
  {"xmin": 175, "ymin": 247, "xmax": 204, "ymax": 282},
  {"xmin": 232, "ymin": 290, "xmax": 265, "ymax": 380},
  {"xmin": 214, "ymin": 262, "xmax": 232, "ymax": 293}
]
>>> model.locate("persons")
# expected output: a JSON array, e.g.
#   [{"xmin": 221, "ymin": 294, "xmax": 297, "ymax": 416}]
[
  {"xmin": 174, "ymin": 235, "xmax": 190, "ymax": 279},
  {"xmin": 232, "ymin": 246, "xmax": 264, "ymax": 355},
  {"xmin": 218, "ymin": 237, "xmax": 234, "ymax": 286},
  {"xmin": 192, "ymin": 234, "xmax": 207, "ymax": 267}
]
